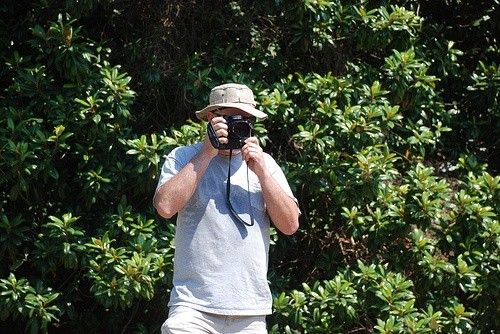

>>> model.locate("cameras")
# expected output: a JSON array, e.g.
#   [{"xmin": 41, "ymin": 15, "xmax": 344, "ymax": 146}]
[{"xmin": 212, "ymin": 114, "xmax": 256, "ymax": 150}]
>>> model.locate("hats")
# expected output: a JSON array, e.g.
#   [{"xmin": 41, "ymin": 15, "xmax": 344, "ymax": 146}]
[{"xmin": 194, "ymin": 82, "xmax": 268, "ymax": 119}]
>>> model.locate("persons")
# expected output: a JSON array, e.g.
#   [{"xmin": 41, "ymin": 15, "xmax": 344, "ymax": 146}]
[{"xmin": 153, "ymin": 83, "xmax": 302, "ymax": 334}]
